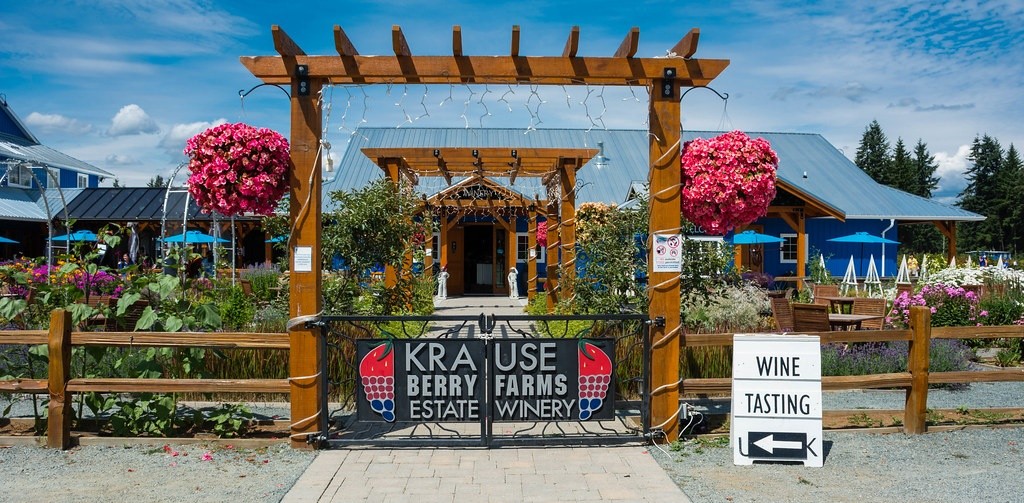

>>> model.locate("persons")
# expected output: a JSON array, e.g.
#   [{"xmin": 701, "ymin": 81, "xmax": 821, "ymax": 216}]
[
  {"xmin": 907, "ymin": 253, "xmax": 920, "ymax": 277},
  {"xmin": 978, "ymin": 255, "xmax": 1022, "ymax": 272},
  {"xmin": 0, "ymin": 241, "xmax": 241, "ymax": 285}
]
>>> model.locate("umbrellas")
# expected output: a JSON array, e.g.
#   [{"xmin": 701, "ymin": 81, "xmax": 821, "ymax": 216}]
[
  {"xmin": 826, "ymin": 231, "xmax": 902, "ymax": 276},
  {"xmin": 159, "ymin": 231, "xmax": 229, "ymax": 243},
  {"xmin": 46, "ymin": 230, "xmax": 104, "ymax": 242},
  {"xmin": 0, "ymin": 237, "xmax": 19, "ymax": 243},
  {"xmin": 726, "ymin": 230, "xmax": 787, "ymax": 272}
]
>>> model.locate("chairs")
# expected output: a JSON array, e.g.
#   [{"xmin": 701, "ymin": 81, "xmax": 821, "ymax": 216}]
[
  {"xmin": 771, "ymin": 297, "xmax": 795, "ymax": 334},
  {"xmin": 811, "ymin": 283, "xmax": 842, "ymax": 316},
  {"xmin": 790, "ymin": 302, "xmax": 831, "ymax": 334},
  {"xmin": 851, "ymin": 296, "xmax": 886, "ymax": 331}
]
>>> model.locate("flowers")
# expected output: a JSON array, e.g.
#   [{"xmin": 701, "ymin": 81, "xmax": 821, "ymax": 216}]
[
  {"xmin": 680, "ymin": 133, "xmax": 779, "ymax": 235},
  {"xmin": 187, "ymin": 122, "xmax": 291, "ymax": 221}
]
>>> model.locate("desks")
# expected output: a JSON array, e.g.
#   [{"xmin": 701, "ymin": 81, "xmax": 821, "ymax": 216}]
[
  {"xmin": 815, "ymin": 295, "xmax": 860, "ymax": 316},
  {"xmin": 827, "ymin": 312, "xmax": 883, "ymax": 346}
]
